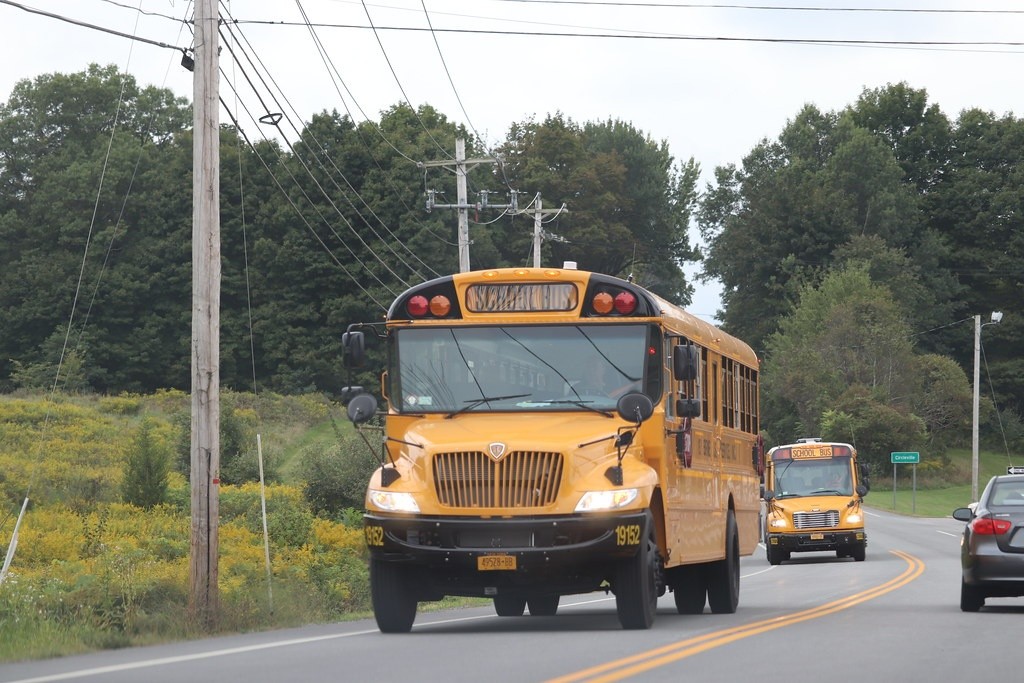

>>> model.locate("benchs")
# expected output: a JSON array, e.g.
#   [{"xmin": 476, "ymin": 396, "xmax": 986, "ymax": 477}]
[{"xmin": 775, "ymin": 477, "xmax": 806, "ymax": 493}]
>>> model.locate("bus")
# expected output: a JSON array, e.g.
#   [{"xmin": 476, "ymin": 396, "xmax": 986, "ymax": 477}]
[
  {"xmin": 339, "ymin": 264, "xmax": 764, "ymax": 633},
  {"xmin": 762, "ymin": 436, "xmax": 870, "ymax": 565}
]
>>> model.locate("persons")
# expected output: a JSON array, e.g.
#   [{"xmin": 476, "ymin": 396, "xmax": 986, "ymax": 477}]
[
  {"xmin": 568, "ymin": 355, "xmax": 621, "ymax": 400},
  {"xmin": 826, "ymin": 470, "xmax": 846, "ymax": 487}
]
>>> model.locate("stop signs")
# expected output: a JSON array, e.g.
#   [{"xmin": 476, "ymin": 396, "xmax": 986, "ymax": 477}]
[{"xmin": 683, "ymin": 417, "xmax": 692, "ymax": 467}]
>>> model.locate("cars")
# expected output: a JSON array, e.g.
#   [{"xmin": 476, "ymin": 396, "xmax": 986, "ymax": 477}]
[{"xmin": 951, "ymin": 465, "xmax": 1024, "ymax": 613}]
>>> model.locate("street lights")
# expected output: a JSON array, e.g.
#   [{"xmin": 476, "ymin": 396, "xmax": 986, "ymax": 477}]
[{"xmin": 973, "ymin": 310, "xmax": 1005, "ymax": 506}]
